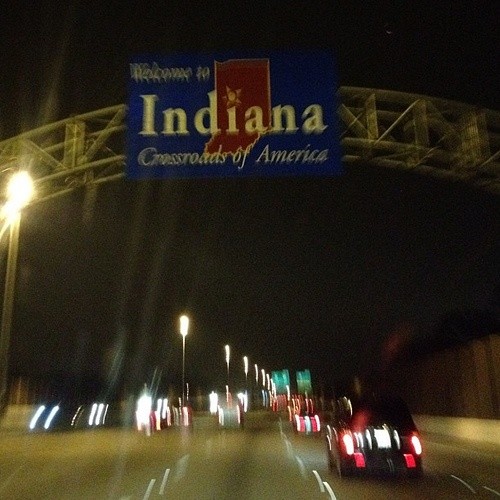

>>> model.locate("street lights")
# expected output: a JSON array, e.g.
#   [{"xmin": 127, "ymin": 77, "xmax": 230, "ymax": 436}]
[
  {"xmin": 241, "ymin": 356, "xmax": 272, "ymax": 412},
  {"xmin": 225, "ymin": 345, "xmax": 231, "ymax": 390},
  {"xmin": 0, "ymin": 168, "xmax": 35, "ymax": 420},
  {"xmin": 178, "ymin": 315, "xmax": 190, "ymax": 427}
]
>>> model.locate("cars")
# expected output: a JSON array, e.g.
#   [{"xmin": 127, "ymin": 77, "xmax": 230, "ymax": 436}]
[
  {"xmin": 326, "ymin": 402, "xmax": 424, "ymax": 479},
  {"xmin": 137, "ymin": 390, "xmax": 352, "ymax": 440}
]
